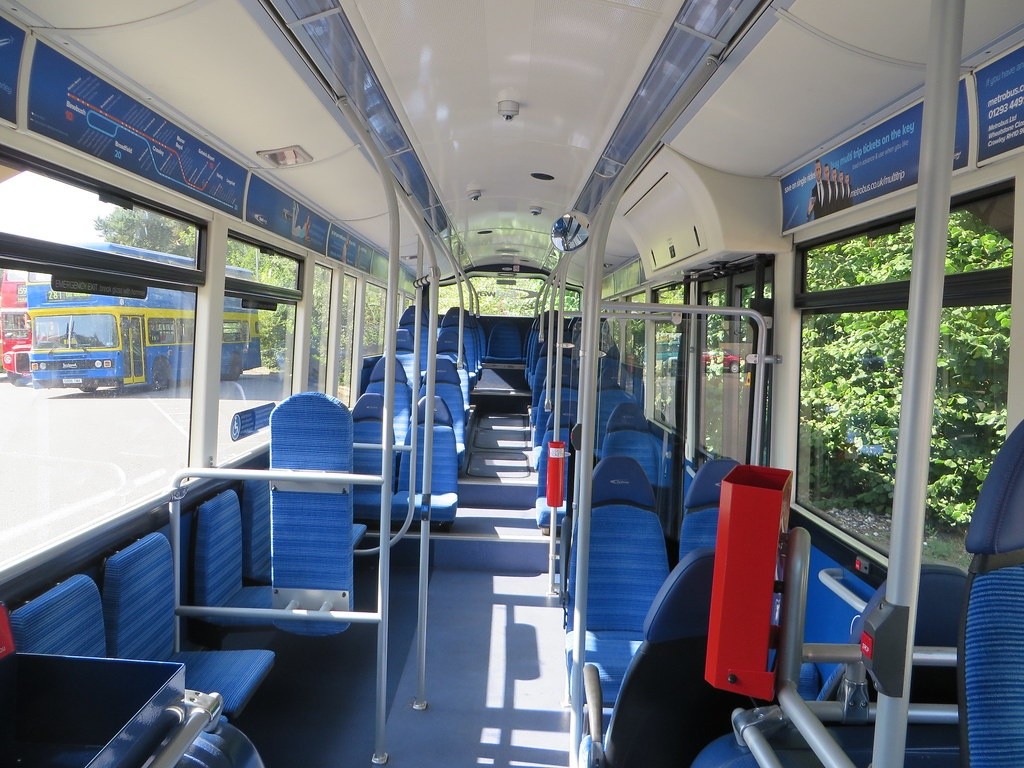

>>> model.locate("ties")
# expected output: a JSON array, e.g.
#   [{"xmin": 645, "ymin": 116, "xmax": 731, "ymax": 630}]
[{"xmin": 819, "ymin": 183, "xmax": 822, "ymax": 205}]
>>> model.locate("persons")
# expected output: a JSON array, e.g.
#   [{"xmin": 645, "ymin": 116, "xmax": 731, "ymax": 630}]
[
  {"xmin": 807, "ymin": 159, "xmax": 852, "ymax": 224},
  {"xmin": 292, "ymin": 200, "xmax": 311, "ymax": 242}
]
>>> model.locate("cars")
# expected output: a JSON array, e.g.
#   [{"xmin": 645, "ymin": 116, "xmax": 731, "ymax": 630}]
[
  {"xmin": 656, "ymin": 341, "xmax": 680, "ymax": 360},
  {"xmin": 699, "ymin": 347, "xmax": 741, "ymax": 374}
]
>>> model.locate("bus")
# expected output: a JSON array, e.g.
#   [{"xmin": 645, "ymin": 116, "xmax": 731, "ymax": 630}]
[
  {"xmin": 0, "ymin": 268, "xmax": 32, "ymax": 386},
  {"xmin": 30, "ymin": 242, "xmax": 261, "ymax": 393}
]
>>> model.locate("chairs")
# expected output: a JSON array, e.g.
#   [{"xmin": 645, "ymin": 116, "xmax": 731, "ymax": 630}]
[{"xmin": 958, "ymin": 414, "xmax": 1024, "ymax": 767}]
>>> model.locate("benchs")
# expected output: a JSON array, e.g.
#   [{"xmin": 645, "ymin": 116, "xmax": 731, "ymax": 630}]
[
  {"xmin": 192, "ymin": 466, "xmax": 367, "ymax": 630},
  {"xmin": 353, "ymin": 305, "xmax": 965, "ymax": 768},
  {"xmin": 15, "ymin": 532, "xmax": 276, "ymax": 719}
]
